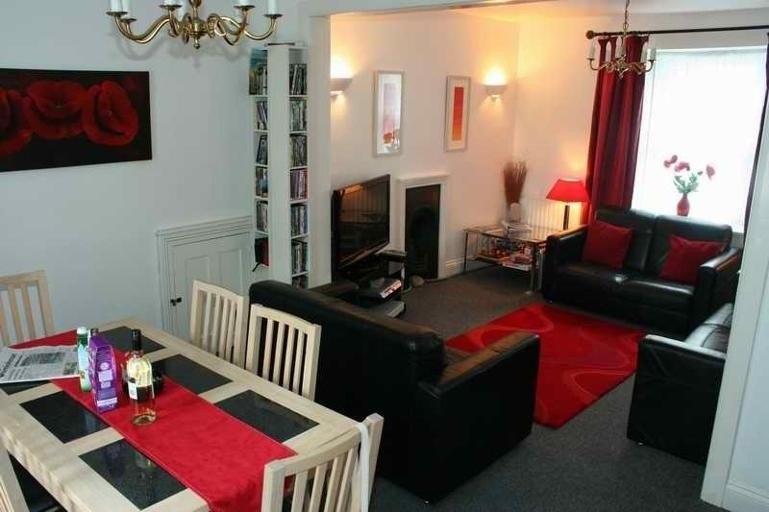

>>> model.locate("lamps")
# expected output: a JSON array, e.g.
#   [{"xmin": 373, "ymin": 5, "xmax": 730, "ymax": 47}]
[
  {"xmin": 546, "ymin": 178, "xmax": 588, "ymax": 229},
  {"xmin": 105, "ymin": 0, "xmax": 281, "ymax": 49},
  {"xmin": 330, "ymin": 58, "xmax": 354, "ymax": 94},
  {"xmin": 585, "ymin": 0, "xmax": 656, "ymax": 80},
  {"xmin": 482, "ymin": 70, "xmax": 509, "ymax": 97}
]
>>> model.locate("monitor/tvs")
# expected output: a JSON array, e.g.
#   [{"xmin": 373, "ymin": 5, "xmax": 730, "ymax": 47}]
[{"xmin": 331, "ymin": 174, "xmax": 390, "ymax": 272}]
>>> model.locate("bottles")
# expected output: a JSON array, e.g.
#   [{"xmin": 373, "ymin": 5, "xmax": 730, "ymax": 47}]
[
  {"xmin": 76, "ymin": 327, "xmax": 99, "ymax": 392},
  {"xmin": 124, "ymin": 329, "xmax": 156, "ymax": 425}
]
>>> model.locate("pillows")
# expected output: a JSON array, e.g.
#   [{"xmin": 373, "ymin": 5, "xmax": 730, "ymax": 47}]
[
  {"xmin": 582, "ymin": 220, "xmax": 633, "ymax": 271},
  {"xmin": 661, "ymin": 232, "xmax": 729, "ymax": 284}
]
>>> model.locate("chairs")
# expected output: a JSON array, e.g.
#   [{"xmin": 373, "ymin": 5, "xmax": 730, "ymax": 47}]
[
  {"xmin": 190, "ymin": 279, "xmax": 249, "ymax": 369},
  {"xmin": 0, "ymin": 268, "xmax": 56, "ymax": 344},
  {"xmin": 261, "ymin": 413, "xmax": 383, "ymax": 512},
  {"xmin": 246, "ymin": 303, "xmax": 322, "ymax": 400}
]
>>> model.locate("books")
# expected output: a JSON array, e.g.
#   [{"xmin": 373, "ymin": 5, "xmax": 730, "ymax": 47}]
[
  {"xmin": 501, "ymin": 218, "xmax": 532, "ymax": 234},
  {"xmin": 475, "ymin": 252, "xmax": 532, "ymax": 271}
]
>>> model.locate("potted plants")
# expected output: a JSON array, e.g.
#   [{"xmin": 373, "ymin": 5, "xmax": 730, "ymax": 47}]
[{"xmin": 503, "ymin": 161, "xmax": 528, "ymax": 223}]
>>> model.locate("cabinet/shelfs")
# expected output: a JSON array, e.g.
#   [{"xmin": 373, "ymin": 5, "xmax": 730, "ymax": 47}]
[{"xmin": 249, "ymin": 45, "xmax": 312, "ymax": 290}]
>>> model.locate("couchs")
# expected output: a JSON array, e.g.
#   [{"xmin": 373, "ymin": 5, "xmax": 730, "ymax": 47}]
[
  {"xmin": 626, "ymin": 269, "xmax": 743, "ymax": 465},
  {"xmin": 251, "ymin": 280, "xmax": 541, "ymax": 505},
  {"xmin": 541, "ymin": 205, "xmax": 742, "ymax": 326}
]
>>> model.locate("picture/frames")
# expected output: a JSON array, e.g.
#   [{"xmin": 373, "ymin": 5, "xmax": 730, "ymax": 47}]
[
  {"xmin": 445, "ymin": 75, "xmax": 472, "ymax": 152},
  {"xmin": 372, "ymin": 68, "xmax": 406, "ymax": 157}
]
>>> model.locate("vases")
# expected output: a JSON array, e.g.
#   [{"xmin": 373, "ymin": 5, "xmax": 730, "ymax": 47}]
[{"xmin": 677, "ymin": 194, "xmax": 690, "ymax": 217}]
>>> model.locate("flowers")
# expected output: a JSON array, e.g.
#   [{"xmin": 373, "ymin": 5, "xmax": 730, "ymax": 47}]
[{"xmin": 665, "ymin": 155, "xmax": 715, "ymax": 196}]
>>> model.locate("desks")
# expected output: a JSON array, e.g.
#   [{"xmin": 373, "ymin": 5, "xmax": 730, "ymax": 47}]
[{"xmin": 338, "ymin": 260, "xmax": 407, "ymax": 302}]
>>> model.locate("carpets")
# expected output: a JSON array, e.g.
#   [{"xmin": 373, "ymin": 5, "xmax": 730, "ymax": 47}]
[{"xmin": 444, "ymin": 299, "xmax": 661, "ymax": 431}]
